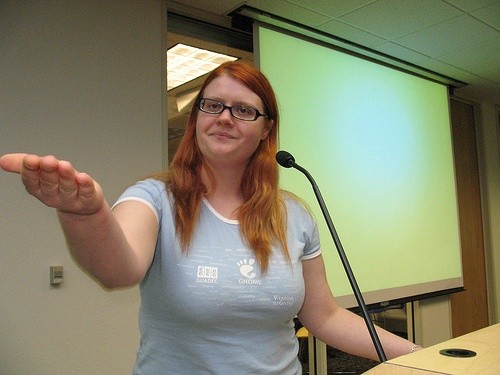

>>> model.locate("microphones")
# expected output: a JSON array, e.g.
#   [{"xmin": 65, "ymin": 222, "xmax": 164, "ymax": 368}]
[{"xmin": 276, "ymin": 150, "xmax": 386, "ymax": 363}]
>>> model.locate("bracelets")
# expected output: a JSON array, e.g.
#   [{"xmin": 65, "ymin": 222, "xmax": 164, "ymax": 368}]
[{"xmin": 409, "ymin": 344, "xmax": 421, "ymax": 353}]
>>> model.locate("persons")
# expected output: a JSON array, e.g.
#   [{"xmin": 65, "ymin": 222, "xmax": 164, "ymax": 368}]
[{"xmin": 0, "ymin": 60, "xmax": 423, "ymax": 375}]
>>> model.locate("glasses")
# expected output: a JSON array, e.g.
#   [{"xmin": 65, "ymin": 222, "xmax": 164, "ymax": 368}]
[{"xmin": 197, "ymin": 97, "xmax": 269, "ymax": 121}]
